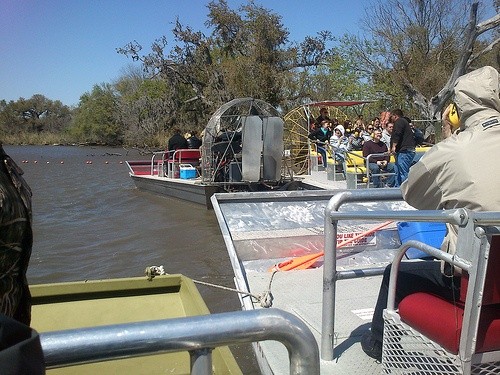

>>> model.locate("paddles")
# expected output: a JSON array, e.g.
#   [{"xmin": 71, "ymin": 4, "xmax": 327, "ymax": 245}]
[{"xmin": 267, "ymin": 219, "xmax": 395, "ymax": 273}]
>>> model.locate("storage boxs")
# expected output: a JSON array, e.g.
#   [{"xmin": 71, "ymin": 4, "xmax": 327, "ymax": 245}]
[{"xmin": 179, "ymin": 164, "xmax": 196, "ymax": 178}]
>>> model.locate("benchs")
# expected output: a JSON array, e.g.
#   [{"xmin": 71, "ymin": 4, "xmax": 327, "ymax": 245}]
[
  {"xmin": 382, "ymin": 212, "xmax": 500, "ymax": 375},
  {"xmin": 309, "ymin": 146, "xmax": 432, "ymax": 189}
]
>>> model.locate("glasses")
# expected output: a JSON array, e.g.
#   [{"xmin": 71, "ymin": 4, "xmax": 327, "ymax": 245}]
[{"xmin": 368, "ymin": 128, "xmax": 374, "ymax": 130}]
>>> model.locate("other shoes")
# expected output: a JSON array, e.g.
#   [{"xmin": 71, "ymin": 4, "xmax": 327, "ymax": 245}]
[{"xmin": 360, "ymin": 333, "xmax": 397, "ymax": 366}]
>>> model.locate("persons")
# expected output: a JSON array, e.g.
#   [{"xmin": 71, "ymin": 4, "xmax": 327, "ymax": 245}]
[
  {"xmin": 162, "ymin": 128, "xmax": 201, "ymax": 176},
  {"xmin": 0, "ymin": 142, "xmax": 35, "ymax": 350},
  {"xmin": 360, "ymin": 65, "xmax": 500, "ymax": 363},
  {"xmin": 302, "ymin": 107, "xmax": 423, "ymax": 189}
]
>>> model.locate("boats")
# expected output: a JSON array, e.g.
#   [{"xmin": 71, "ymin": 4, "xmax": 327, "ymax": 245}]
[
  {"xmin": 210, "ymin": 185, "xmax": 500, "ymax": 374},
  {"xmin": 126, "ymin": 96, "xmax": 304, "ymax": 211}
]
